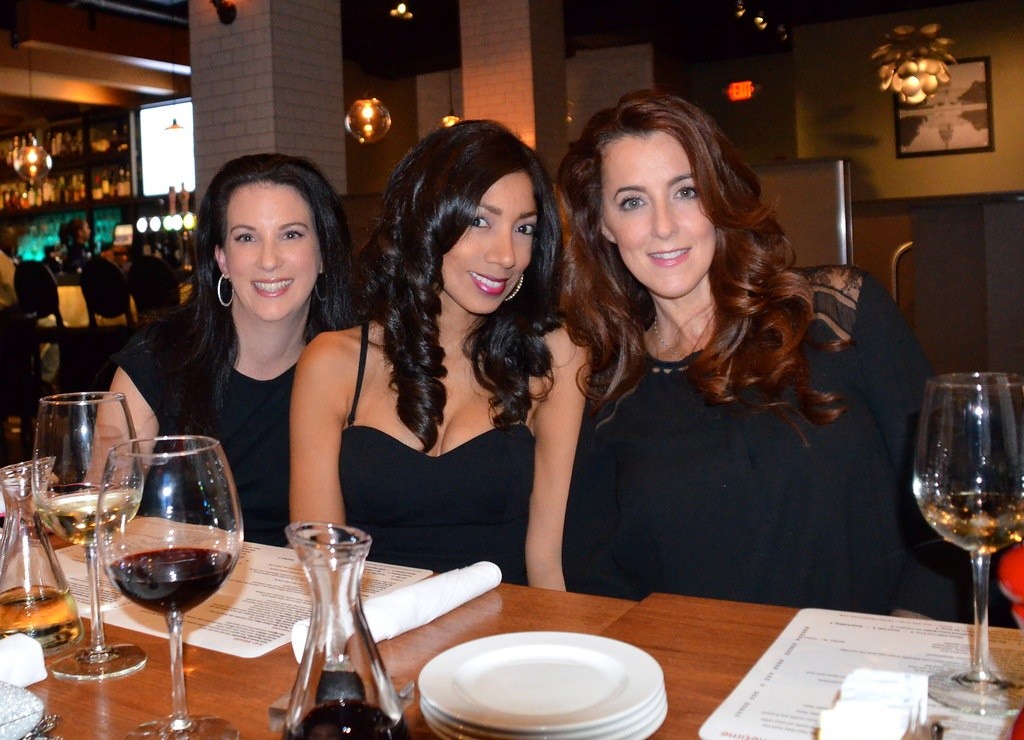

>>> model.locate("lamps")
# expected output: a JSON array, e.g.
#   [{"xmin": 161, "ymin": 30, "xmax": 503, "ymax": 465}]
[
  {"xmin": 442, "ymin": 70, "xmax": 459, "ymax": 127},
  {"xmin": 13, "ymin": 49, "xmax": 53, "ymax": 183},
  {"xmin": 870, "ymin": 22, "xmax": 957, "ymax": 107},
  {"xmin": 344, "ymin": 72, "xmax": 391, "ymax": 142}
]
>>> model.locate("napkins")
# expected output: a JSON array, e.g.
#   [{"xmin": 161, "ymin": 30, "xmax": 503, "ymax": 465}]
[{"xmin": 290, "ymin": 560, "xmax": 503, "ymax": 664}]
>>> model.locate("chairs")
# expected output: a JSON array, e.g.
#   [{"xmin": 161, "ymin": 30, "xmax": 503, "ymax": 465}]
[{"xmin": 13, "ymin": 256, "xmax": 180, "ymax": 444}]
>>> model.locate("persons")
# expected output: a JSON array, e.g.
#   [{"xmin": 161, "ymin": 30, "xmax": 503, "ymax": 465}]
[
  {"xmin": 288, "ymin": 119, "xmax": 589, "ymax": 595},
  {"xmin": 0, "ymin": 220, "xmax": 133, "ymax": 313},
  {"xmin": 79, "ymin": 152, "xmax": 352, "ymax": 552},
  {"xmin": 563, "ymin": 89, "xmax": 970, "ymax": 623}
]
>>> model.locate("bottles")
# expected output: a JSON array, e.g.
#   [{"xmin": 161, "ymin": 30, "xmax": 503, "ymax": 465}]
[
  {"xmin": 284, "ymin": 520, "xmax": 405, "ymax": 740},
  {"xmin": 0, "ymin": 457, "xmax": 84, "ymax": 657}
]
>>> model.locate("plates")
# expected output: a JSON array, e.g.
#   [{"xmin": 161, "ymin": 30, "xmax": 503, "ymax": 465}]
[{"xmin": 419, "ymin": 631, "xmax": 669, "ymax": 740}]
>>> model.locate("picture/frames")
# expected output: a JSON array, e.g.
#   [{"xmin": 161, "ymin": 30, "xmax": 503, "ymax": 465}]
[{"xmin": 892, "ymin": 55, "xmax": 995, "ymax": 158}]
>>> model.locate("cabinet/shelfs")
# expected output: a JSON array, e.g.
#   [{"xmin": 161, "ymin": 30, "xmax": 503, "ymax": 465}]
[{"xmin": 0, "ymin": 109, "xmax": 195, "ymax": 255}]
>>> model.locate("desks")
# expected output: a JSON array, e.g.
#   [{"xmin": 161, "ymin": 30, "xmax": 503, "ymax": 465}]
[{"xmin": 0, "ymin": 517, "xmax": 798, "ymax": 740}]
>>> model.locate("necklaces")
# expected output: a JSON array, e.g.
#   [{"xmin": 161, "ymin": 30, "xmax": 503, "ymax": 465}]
[{"xmin": 654, "ymin": 317, "xmax": 683, "ymax": 357}]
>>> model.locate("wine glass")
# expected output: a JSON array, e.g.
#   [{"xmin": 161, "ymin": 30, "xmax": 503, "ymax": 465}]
[
  {"xmin": 33, "ymin": 392, "xmax": 146, "ymax": 680},
  {"xmin": 917, "ymin": 375, "xmax": 1024, "ymax": 720},
  {"xmin": 97, "ymin": 436, "xmax": 245, "ymax": 740}
]
